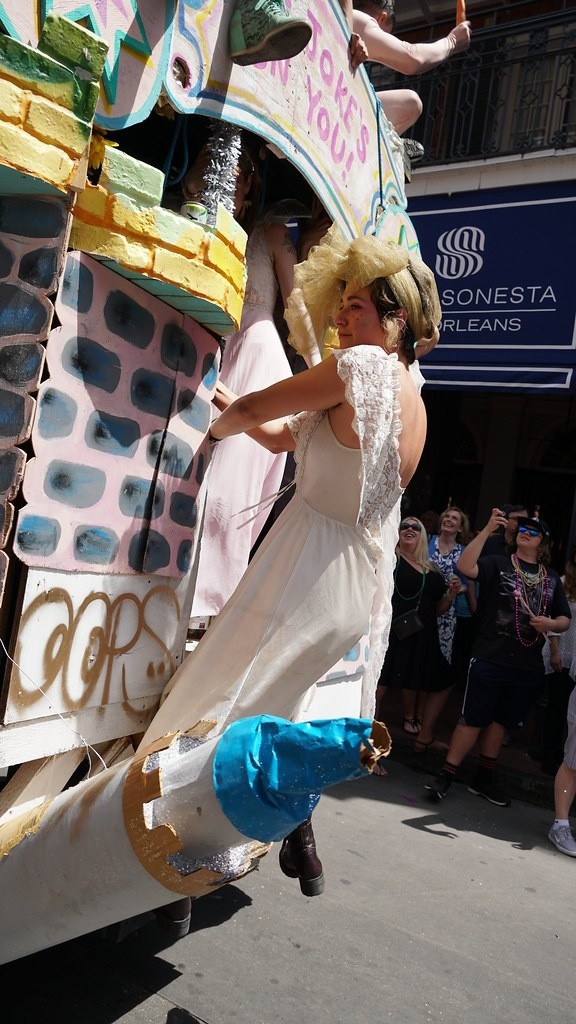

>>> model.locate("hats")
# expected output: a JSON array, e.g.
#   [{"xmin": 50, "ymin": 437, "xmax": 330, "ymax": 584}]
[
  {"xmin": 517, "ymin": 517, "xmax": 551, "ymax": 538},
  {"xmin": 283, "ymin": 218, "xmax": 442, "ymax": 359}
]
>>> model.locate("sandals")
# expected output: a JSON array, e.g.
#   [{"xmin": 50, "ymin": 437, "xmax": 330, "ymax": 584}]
[{"xmin": 415, "ymin": 736, "xmax": 450, "ymax": 752}]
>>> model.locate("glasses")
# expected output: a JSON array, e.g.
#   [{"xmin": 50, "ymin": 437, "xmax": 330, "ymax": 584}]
[
  {"xmin": 399, "ymin": 522, "xmax": 421, "ymax": 531},
  {"xmin": 509, "ymin": 516, "xmax": 517, "ymax": 520},
  {"xmin": 519, "ymin": 524, "xmax": 538, "ymax": 536}
]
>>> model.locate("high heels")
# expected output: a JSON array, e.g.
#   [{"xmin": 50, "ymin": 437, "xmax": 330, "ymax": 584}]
[
  {"xmin": 279, "ymin": 813, "xmax": 324, "ymax": 896},
  {"xmin": 157, "ymin": 897, "xmax": 191, "ymax": 936}
]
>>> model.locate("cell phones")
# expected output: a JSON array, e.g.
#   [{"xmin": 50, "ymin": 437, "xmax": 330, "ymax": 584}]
[{"xmin": 497, "ymin": 504, "xmax": 511, "ymax": 522}]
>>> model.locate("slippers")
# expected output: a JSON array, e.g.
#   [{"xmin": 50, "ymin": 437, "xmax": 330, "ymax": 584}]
[
  {"xmin": 373, "ymin": 760, "xmax": 388, "ymax": 776},
  {"xmin": 402, "ymin": 718, "xmax": 423, "ymax": 735}
]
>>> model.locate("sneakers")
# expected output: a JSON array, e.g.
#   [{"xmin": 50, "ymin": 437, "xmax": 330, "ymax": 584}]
[
  {"xmin": 424, "ymin": 769, "xmax": 454, "ymax": 800},
  {"xmin": 468, "ymin": 775, "xmax": 512, "ymax": 807},
  {"xmin": 548, "ymin": 821, "xmax": 576, "ymax": 856}
]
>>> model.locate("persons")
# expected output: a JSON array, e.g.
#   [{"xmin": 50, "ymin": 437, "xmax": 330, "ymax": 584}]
[
  {"xmin": 423, "ymin": 508, "xmax": 572, "ymax": 805},
  {"xmin": 350, "ymin": 0, "xmax": 471, "ymax": 164},
  {"xmin": 466, "ymin": 505, "xmax": 529, "ymax": 644},
  {"xmin": 542, "ymin": 556, "xmax": 576, "ymax": 859},
  {"xmin": 181, "ymin": 145, "xmax": 320, "ymax": 618},
  {"xmin": 228, "ymin": 0, "xmax": 313, "ymax": 66},
  {"xmin": 338, "ymin": 0, "xmax": 369, "ymax": 70},
  {"xmin": 134, "ymin": 219, "xmax": 442, "ymax": 938},
  {"xmin": 371, "ymin": 516, "xmax": 462, "ymax": 775},
  {"xmin": 427, "ymin": 506, "xmax": 468, "ymax": 645}
]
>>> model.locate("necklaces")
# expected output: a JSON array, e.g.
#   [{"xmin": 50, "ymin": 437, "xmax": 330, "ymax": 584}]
[
  {"xmin": 511, "ymin": 554, "xmax": 548, "ymax": 647},
  {"xmin": 395, "ymin": 559, "xmax": 426, "ymax": 600}
]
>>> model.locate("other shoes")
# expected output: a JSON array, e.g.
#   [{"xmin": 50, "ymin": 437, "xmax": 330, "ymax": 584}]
[
  {"xmin": 400, "ymin": 138, "xmax": 424, "ymax": 162},
  {"xmin": 502, "ymin": 734, "xmax": 511, "ymax": 746}
]
